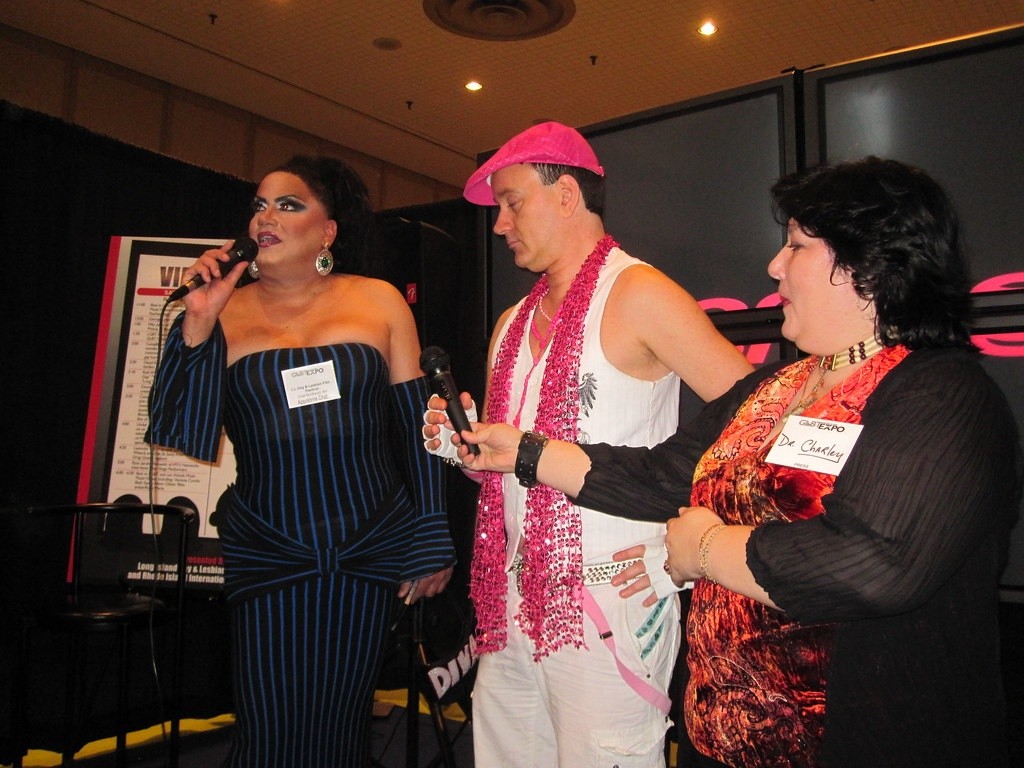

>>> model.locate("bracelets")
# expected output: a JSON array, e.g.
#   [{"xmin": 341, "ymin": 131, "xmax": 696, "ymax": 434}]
[
  {"xmin": 515, "ymin": 430, "xmax": 550, "ymax": 488},
  {"xmin": 698, "ymin": 524, "xmax": 727, "ymax": 584}
]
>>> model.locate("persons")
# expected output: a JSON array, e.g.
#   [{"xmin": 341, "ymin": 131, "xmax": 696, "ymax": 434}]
[
  {"xmin": 142, "ymin": 156, "xmax": 457, "ymax": 768},
  {"xmin": 443, "ymin": 157, "xmax": 1023, "ymax": 768},
  {"xmin": 422, "ymin": 122, "xmax": 757, "ymax": 768}
]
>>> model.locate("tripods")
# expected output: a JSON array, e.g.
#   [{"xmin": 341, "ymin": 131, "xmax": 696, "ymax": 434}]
[{"xmin": 381, "ymin": 595, "xmax": 474, "ymax": 768}]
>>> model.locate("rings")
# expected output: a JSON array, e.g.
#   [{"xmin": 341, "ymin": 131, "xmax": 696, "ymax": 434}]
[{"xmin": 664, "ymin": 561, "xmax": 671, "ymax": 574}]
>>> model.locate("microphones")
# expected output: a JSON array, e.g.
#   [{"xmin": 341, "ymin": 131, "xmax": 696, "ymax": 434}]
[
  {"xmin": 166, "ymin": 238, "xmax": 259, "ymax": 303},
  {"xmin": 420, "ymin": 346, "xmax": 482, "ymax": 456}
]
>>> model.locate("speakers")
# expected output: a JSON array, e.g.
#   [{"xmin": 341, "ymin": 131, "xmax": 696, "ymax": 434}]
[{"xmin": 361, "ymin": 218, "xmax": 465, "ymax": 356}]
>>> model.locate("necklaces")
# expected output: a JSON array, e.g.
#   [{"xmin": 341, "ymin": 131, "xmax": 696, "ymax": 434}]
[
  {"xmin": 539, "ymin": 287, "xmax": 553, "ymax": 322},
  {"xmin": 782, "ymin": 325, "xmax": 900, "ymax": 423},
  {"xmin": 514, "ymin": 301, "xmax": 563, "ymax": 430}
]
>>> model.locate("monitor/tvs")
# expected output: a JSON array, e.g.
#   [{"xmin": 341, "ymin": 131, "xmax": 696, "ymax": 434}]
[{"xmin": 477, "ymin": 24, "xmax": 1024, "ymax": 608}]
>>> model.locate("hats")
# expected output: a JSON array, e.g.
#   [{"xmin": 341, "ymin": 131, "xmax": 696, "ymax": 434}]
[{"xmin": 463, "ymin": 122, "xmax": 605, "ymax": 206}]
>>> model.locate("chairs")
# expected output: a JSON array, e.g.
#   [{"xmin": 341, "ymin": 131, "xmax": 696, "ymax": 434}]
[{"xmin": 12, "ymin": 502, "xmax": 196, "ymax": 768}]
